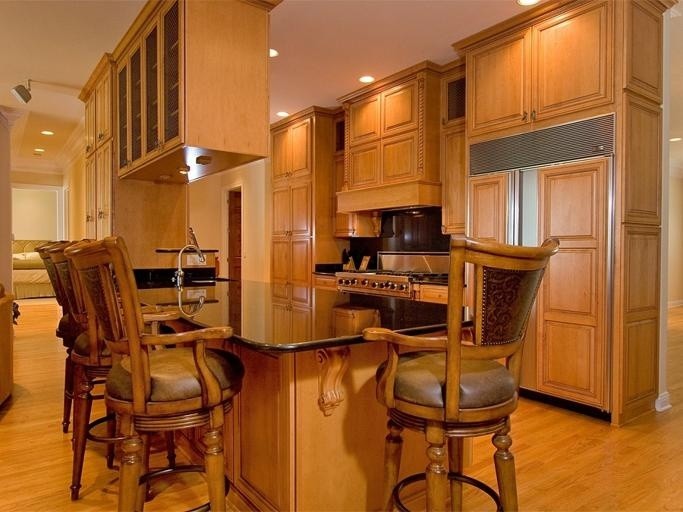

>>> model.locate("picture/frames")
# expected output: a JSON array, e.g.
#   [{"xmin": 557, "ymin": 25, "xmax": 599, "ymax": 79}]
[{"xmin": 137, "ymin": 278, "xmax": 470, "ymax": 512}]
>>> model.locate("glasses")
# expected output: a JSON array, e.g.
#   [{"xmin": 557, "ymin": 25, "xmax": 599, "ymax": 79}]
[{"xmin": 10, "ymin": 79, "xmax": 32, "ymax": 105}]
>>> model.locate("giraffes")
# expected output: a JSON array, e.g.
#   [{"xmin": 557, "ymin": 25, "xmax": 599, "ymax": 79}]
[{"xmin": 335, "ymin": 250, "xmax": 450, "ymax": 299}]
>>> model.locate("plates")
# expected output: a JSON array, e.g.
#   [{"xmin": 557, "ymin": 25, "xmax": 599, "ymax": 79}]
[
  {"xmin": 452, "ymin": 0, "xmax": 677, "ymax": 414},
  {"xmin": 111, "ymin": 1, "xmax": 270, "ymax": 186},
  {"xmin": 271, "ymin": 236, "xmax": 313, "ymax": 287},
  {"xmin": 271, "ymin": 180, "xmax": 313, "ymax": 237},
  {"xmin": 335, "ymin": 59, "xmax": 441, "ymax": 214},
  {"xmin": 271, "ymin": 117, "xmax": 313, "ymax": 183},
  {"xmin": 271, "ymin": 283, "xmax": 312, "ymax": 308},
  {"xmin": 271, "ymin": 303, "xmax": 312, "ymax": 345},
  {"xmin": 78, "ymin": 52, "xmax": 189, "ymax": 270}
]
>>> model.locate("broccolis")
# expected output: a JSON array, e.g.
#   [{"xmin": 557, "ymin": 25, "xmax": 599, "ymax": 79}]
[{"xmin": 465, "ymin": 114, "xmax": 619, "ymax": 427}]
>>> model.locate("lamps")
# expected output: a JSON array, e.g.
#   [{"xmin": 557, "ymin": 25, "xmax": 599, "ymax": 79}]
[
  {"xmin": 33, "ymin": 241, "xmax": 90, "ymax": 434},
  {"xmin": 63, "ymin": 237, "xmax": 246, "ymax": 512},
  {"xmin": 362, "ymin": 235, "xmax": 561, "ymax": 512},
  {"xmin": 44, "ymin": 238, "xmax": 177, "ymax": 501}
]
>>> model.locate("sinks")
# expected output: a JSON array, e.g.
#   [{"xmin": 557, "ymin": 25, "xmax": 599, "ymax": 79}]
[{"xmin": 12, "ymin": 240, "xmax": 56, "ymax": 299}]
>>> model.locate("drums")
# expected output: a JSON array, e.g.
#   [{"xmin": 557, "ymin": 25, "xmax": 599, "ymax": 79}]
[{"xmin": 342, "ymin": 248, "xmax": 350, "ymax": 264}]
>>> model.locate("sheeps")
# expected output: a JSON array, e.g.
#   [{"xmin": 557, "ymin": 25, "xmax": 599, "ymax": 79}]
[{"xmin": 177, "ymin": 244, "xmax": 206, "ymax": 276}]
